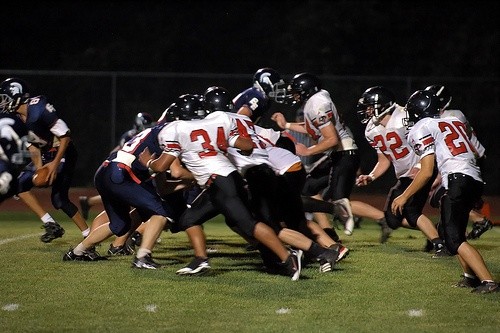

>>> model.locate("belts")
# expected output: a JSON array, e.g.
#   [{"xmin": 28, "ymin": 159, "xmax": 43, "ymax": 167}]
[{"xmin": 102, "ymin": 160, "xmax": 141, "ymax": 184}]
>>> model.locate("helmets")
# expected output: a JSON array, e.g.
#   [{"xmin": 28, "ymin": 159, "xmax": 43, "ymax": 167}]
[
  {"xmin": 135, "ymin": 112, "xmax": 153, "ymax": 131},
  {"xmin": 203, "ymin": 86, "xmax": 233, "ymax": 115},
  {"xmin": 0, "ymin": 78, "xmax": 30, "ymax": 112},
  {"xmin": 424, "ymin": 85, "xmax": 452, "ymax": 111},
  {"xmin": 164, "ymin": 95, "xmax": 207, "ymax": 123},
  {"xmin": 363, "ymin": 86, "xmax": 396, "ymax": 123},
  {"xmin": 357, "ymin": 97, "xmax": 374, "ymax": 124},
  {"xmin": 291, "ymin": 73, "xmax": 320, "ymax": 106},
  {"xmin": 406, "ymin": 90, "xmax": 440, "ymax": 126},
  {"xmin": 253, "ymin": 68, "xmax": 285, "ymax": 100}
]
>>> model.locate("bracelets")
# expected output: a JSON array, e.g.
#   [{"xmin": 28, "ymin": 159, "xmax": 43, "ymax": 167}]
[
  {"xmin": 369, "ymin": 174, "xmax": 376, "ymax": 181},
  {"xmin": 286, "ymin": 123, "xmax": 291, "ymax": 129}
]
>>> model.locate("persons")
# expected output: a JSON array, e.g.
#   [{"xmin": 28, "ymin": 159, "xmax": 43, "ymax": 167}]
[
  {"xmin": 0, "ymin": 78, "xmax": 90, "ymax": 239},
  {"xmin": 426, "ymin": 85, "xmax": 493, "ymax": 240},
  {"xmin": 272, "ymin": 73, "xmax": 393, "ymax": 244},
  {"xmin": 63, "ymin": 68, "xmax": 354, "ymax": 281},
  {"xmin": 391, "ymin": 91, "xmax": 500, "ymax": 295},
  {"xmin": 356, "ymin": 86, "xmax": 450, "ymax": 257},
  {"xmin": 0, "ymin": 88, "xmax": 65, "ymax": 243}
]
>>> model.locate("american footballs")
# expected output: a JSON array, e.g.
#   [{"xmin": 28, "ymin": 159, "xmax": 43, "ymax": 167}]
[{"xmin": 31, "ymin": 167, "xmax": 53, "ymax": 188}]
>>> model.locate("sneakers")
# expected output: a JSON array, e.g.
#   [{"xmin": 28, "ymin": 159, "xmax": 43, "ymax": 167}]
[
  {"xmin": 63, "ymin": 248, "xmax": 95, "ymax": 261},
  {"xmin": 451, "ymin": 275, "xmax": 481, "ymax": 288},
  {"xmin": 41, "ymin": 221, "xmax": 65, "ymax": 242},
  {"xmin": 175, "ymin": 258, "xmax": 213, "ymax": 276},
  {"xmin": 132, "ymin": 253, "xmax": 165, "ymax": 269},
  {"xmin": 245, "ymin": 197, "xmax": 363, "ymax": 281},
  {"xmin": 433, "ymin": 239, "xmax": 448, "ymax": 256},
  {"xmin": 376, "ymin": 217, "xmax": 391, "ymax": 243},
  {"xmin": 85, "ymin": 247, "xmax": 107, "ymax": 260},
  {"xmin": 467, "ymin": 216, "xmax": 493, "ymax": 240},
  {"xmin": 470, "ymin": 279, "xmax": 499, "ymax": 294},
  {"xmin": 79, "ymin": 196, "xmax": 90, "ymax": 219},
  {"xmin": 107, "ymin": 228, "xmax": 161, "ymax": 257}
]
